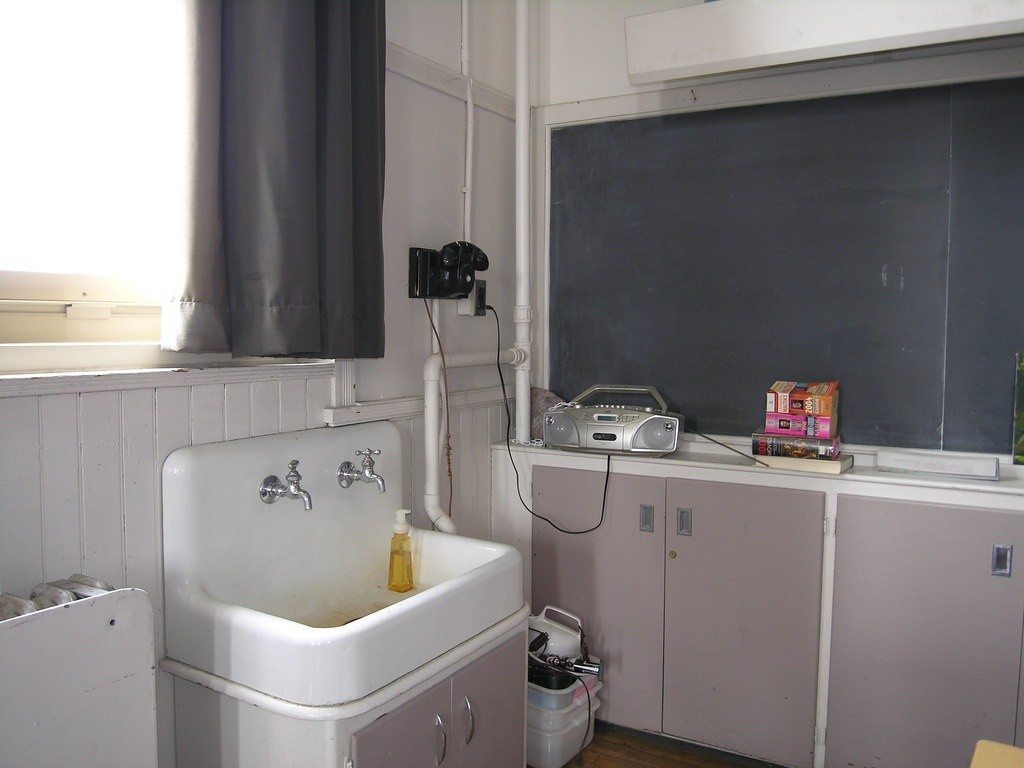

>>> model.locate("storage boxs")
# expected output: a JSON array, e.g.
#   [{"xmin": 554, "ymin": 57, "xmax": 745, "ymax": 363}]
[
  {"xmin": 528, "ymin": 674, "xmax": 597, "ymax": 710},
  {"xmin": 527, "ymin": 697, "xmax": 601, "ymax": 768},
  {"xmin": 527, "ymin": 680, "xmax": 603, "ymax": 732}
]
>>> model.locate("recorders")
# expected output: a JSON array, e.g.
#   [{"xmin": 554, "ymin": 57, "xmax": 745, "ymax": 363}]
[{"xmin": 542, "ymin": 385, "xmax": 685, "ymax": 457}]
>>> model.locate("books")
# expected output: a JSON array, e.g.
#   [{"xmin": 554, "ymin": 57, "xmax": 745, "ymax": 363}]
[{"xmin": 751, "ymin": 380, "xmax": 855, "ymax": 476}]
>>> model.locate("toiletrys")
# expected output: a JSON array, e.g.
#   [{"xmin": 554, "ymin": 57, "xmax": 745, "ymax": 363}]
[{"xmin": 388, "ymin": 507, "xmax": 414, "ymax": 592}]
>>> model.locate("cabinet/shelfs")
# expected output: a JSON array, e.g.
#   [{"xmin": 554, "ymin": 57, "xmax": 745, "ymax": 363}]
[
  {"xmin": 531, "ymin": 464, "xmax": 827, "ymax": 768},
  {"xmin": 823, "ymin": 492, "xmax": 1024, "ymax": 768},
  {"xmin": 349, "ymin": 631, "xmax": 527, "ymax": 768}
]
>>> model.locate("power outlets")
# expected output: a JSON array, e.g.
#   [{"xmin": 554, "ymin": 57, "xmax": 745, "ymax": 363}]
[{"xmin": 457, "ymin": 280, "xmax": 486, "ymax": 317}]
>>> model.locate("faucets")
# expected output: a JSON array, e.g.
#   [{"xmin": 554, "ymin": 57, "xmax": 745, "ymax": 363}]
[
  {"xmin": 335, "ymin": 448, "xmax": 387, "ymax": 493},
  {"xmin": 261, "ymin": 458, "xmax": 314, "ymax": 512}
]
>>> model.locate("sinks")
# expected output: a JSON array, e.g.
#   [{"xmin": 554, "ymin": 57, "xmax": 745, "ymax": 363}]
[{"xmin": 165, "ymin": 527, "xmax": 527, "ymax": 705}]
